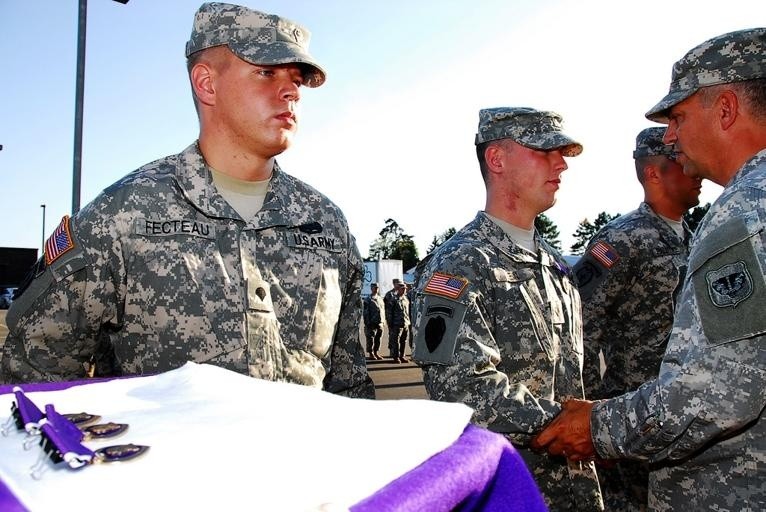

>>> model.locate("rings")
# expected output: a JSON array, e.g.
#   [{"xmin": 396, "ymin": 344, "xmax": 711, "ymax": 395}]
[{"xmin": 560, "ymin": 448, "xmax": 568, "ymax": 457}]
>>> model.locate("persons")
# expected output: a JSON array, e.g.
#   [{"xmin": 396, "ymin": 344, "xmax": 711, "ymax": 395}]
[
  {"xmin": 363, "ymin": 279, "xmax": 413, "ymax": 364},
  {"xmin": 572, "ymin": 127, "xmax": 704, "ymax": 512},
  {"xmin": 0, "ymin": 2, "xmax": 376, "ymax": 398},
  {"xmin": 530, "ymin": 28, "xmax": 766, "ymax": 512},
  {"xmin": 410, "ymin": 107, "xmax": 605, "ymax": 511}
]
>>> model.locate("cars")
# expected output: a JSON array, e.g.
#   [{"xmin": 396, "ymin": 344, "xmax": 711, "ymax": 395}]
[{"xmin": 0, "ymin": 284, "xmax": 18, "ymax": 311}]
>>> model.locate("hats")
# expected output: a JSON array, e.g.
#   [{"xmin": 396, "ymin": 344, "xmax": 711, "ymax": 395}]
[
  {"xmin": 393, "ymin": 279, "xmax": 408, "ymax": 289},
  {"xmin": 645, "ymin": 28, "xmax": 766, "ymax": 124},
  {"xmin": 634, "ymin": 127, "xmax": 676, "ymax": 161},
  {"xmin": 371, "ymin": 284, "xmax": 378, "ymax": 288},
  {"xmin": 185, "ymin": 3, "xmax": 326, "ymax": 87},
  {"xmin": 475, "ymin": 107, "xmax": 583, "ymax": 157}
]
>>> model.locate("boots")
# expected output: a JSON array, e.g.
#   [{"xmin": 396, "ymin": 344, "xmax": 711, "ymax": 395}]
[
  {"xmin": 390, "ymin": 349, "xmax": 408, "ymax": 363},
  {"xmin": 370, "ymin": 350, "xmax": 382, "ymax": 360}
]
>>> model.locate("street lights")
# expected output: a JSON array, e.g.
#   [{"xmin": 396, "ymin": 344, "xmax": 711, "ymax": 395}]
[{"xmin": 39, "ymin": 204, "xmax": 45, "ymax": 254}]
